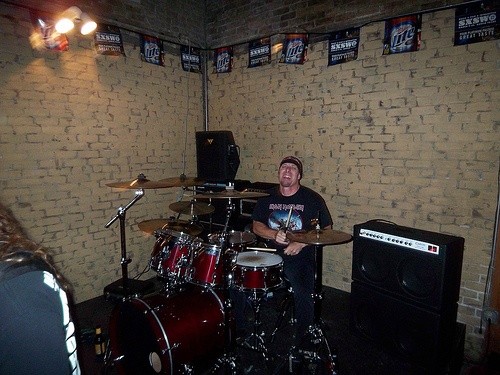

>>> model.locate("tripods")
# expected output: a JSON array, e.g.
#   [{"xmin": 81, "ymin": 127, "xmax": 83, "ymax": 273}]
[{"xmin": 206, "ymin": 244, "xmax": 339, "ymax": 374}]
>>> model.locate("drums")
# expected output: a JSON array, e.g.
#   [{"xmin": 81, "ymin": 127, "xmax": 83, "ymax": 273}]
[
  {"xmin": 231, "ymin": 250, "xmax": 284, "ymax": 292},
  {"xmin": 109, "ymin": 283, "xmax": 226, "ymax": 375},
  {"xmin": 148, "ymin": 229, "xmax": 200, "ymax": 281},
  {"xmin": 208, "ymin": 231, "xmax": 258, "ymax": 253},
  {"xmin": 183, "ymin": 242, "xmax": 234, "ymax": 293}
]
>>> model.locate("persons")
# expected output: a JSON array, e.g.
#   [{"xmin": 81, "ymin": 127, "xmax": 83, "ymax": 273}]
[
  {"xmin": 252, "ymin": 155, "xmax": 333, "ymax": 339},
  {"xmin": 0, "ymin": 203, "xmax": 81, "ymax": 375}
]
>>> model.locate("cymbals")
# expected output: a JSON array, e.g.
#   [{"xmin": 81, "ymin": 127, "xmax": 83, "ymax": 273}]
[
  {"xmin": 170, "ymin": 201, "xmax": 215, "ymax": 216},
  {"xmin": 138, "ymin": 217, "xmax": 204, "ymax": 237},
  {"xmin": 191, "ymin": 189, "xmax": 271, "ymax": 200},
  {"xmin": 286, "ymin": 227, "xmax": 354, "ymax": 247},
  {"xmin": 162, "ymin": 175, "xmax": 205, "ymax": 186},
  {"xmin": 105, "ymin": 177, "xmax": 175, "ymax": 190}
]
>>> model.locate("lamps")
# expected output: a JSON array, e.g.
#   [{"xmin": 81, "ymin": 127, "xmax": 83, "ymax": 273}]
[{"xmin": 37, "ymin": 6, "xmax": 97, "ymax": 41}]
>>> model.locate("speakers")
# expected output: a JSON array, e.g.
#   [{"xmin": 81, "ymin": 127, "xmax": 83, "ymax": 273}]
[
  {"xmin": 346, "ymin": 281, "xmax": 459, "ymax": 375},
  {"xmin": 350, "ymin": 221, "xmax": 466, "ymax": 308},
  {"xmin": 194, "ymin": 129, "xmax": 241, "ymax": 184}
]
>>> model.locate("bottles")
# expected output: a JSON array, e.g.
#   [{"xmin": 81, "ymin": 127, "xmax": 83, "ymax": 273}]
[{"xmin": 94, "ymin": 324, "xmax": 106, "ymax": 360}]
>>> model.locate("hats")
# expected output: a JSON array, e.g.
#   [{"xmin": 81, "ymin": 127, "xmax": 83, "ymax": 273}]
[{"xmin": 279, "ymin": 156, "xmax": 302, "ymax": 175}]
